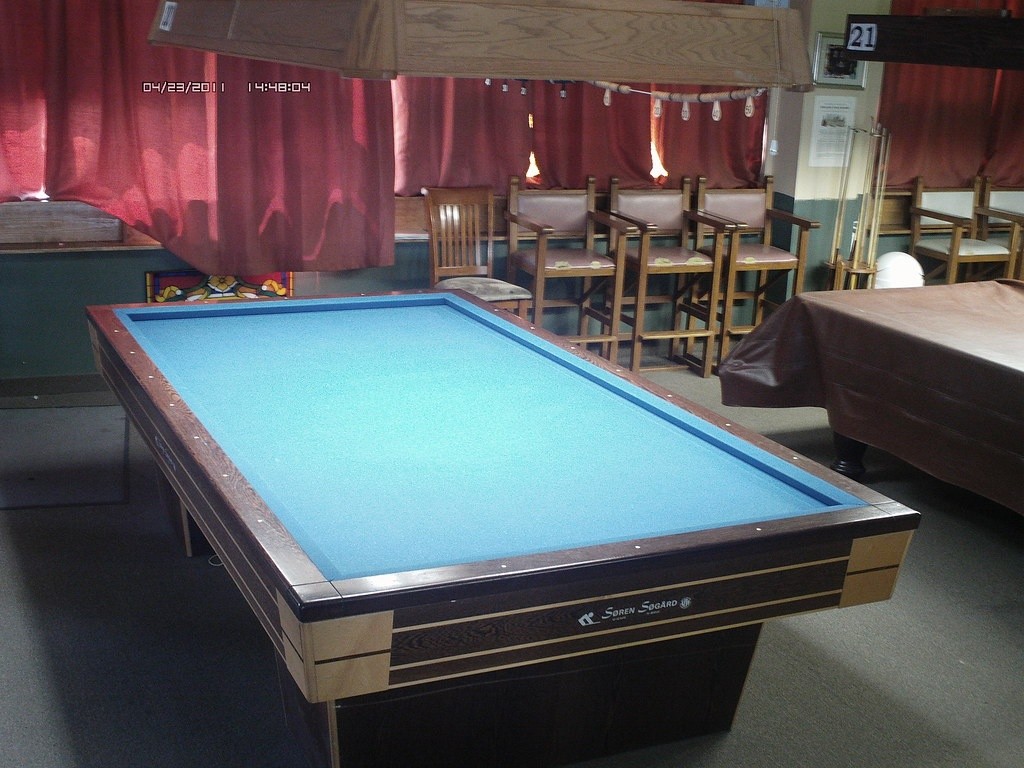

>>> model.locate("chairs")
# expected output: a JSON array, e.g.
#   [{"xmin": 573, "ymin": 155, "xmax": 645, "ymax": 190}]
[
  {"xmin": 909, "ymin": 176, "xmax": 1024, "ymax": 286},
  {"xmin": 426, "ymin": 187, "xmax": 533, "ymax": 322}
]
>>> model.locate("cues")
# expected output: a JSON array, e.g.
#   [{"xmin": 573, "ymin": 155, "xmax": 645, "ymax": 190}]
[{"xmin": 823, "ymin": 115, "xmax": 893, "ymax": 291}]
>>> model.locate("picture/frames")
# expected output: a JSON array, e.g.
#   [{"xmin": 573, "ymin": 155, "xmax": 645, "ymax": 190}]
[{"xmin": 812, "ymin": 31, "xmax": 868, "ymax": 92}]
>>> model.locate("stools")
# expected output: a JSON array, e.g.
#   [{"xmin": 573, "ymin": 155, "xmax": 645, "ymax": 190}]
[
  {"xmin": 503, "ymin": 177, "xmax": 636, "ymax": 367},
  {"xmin": 601, "ymin": 175, "xmax": 736, "ymax": 378},
  {"xmin": 684, "ymin": 171, "xmax": 822, "ymax": 376}
]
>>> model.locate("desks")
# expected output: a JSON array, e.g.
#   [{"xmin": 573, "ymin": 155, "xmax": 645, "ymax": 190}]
[
  {"xmin": 720, "ymin": 271, "xmax": 1024, "ymax": 516},
  {"xmin": 85, "ymin": 287, "xmax": 919, "ymax": 768}
]
